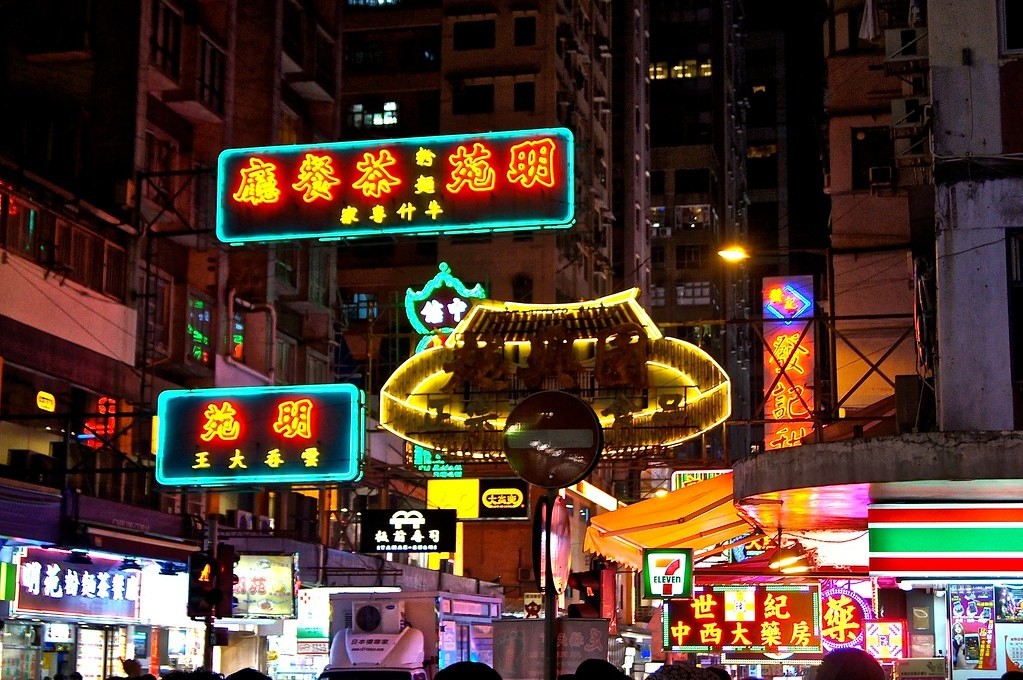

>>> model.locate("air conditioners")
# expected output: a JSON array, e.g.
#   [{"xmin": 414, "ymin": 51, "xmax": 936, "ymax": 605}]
[
  {"xmin": 648, "ymin": 228, "xmax": 657, "ymax": 238},
  {"xmin": 895, "ymin": 374, "xmax": 926, "ymax": 434},
  {"xmin": 884, "ymin": 29, "xmax": 928, "ymax": 56},
  {"xmin": 894, "ymin": 137, "xmax": 932, "ymax": 157},
  {"xmin": 889, "ymin": 96, "xmax": 931, "ymax": 125},
  {"xmin": 658, "ymin": 227, "xmax": 671, "ymax": 237},
  {"xmin": 352, "ymin": 599, "xmax": 405, "ymax": 633}
]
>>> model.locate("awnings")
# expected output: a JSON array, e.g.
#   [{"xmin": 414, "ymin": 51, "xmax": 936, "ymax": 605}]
[{"xmin": 583, "ymin": 470, "xmax": 758, "ymax": 573}]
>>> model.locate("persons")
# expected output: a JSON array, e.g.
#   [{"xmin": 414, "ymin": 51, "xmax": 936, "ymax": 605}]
[{"xmin": 44, "ymin": 647, "xmax": 885, "ymax": 680}]
